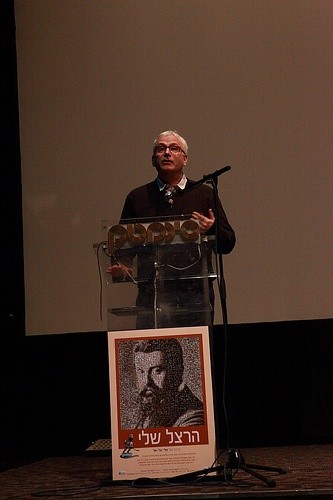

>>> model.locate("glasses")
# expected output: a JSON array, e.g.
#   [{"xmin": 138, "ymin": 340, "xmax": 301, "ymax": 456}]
[{"xmin": 155, "ymin": 146, "xmax": 186, "ymax": 154}]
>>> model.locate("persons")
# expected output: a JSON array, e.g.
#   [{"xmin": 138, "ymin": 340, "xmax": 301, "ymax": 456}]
[
  {"xmin": 131, "ymin": 337, "xmax": 205, "ymax": 429},
  {"xmin": 106, "ymin": 130, "xmax": 236, "ymax": 330}
]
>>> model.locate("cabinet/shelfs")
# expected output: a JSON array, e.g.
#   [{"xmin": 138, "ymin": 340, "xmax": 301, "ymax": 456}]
[{"xmin": 108, "ymin": 235, "xmax": 221, "ymax": 328}]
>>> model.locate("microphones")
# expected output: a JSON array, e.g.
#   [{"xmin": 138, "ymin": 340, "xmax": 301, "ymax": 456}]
[{"xmin": 164, "ymin": 190, "xmax": 173, "ymax": 206}]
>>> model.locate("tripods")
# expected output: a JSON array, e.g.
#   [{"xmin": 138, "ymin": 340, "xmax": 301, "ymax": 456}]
[{"xmin": 162, "ymin": 166, "xmax": 289, "ymax": 489}]
